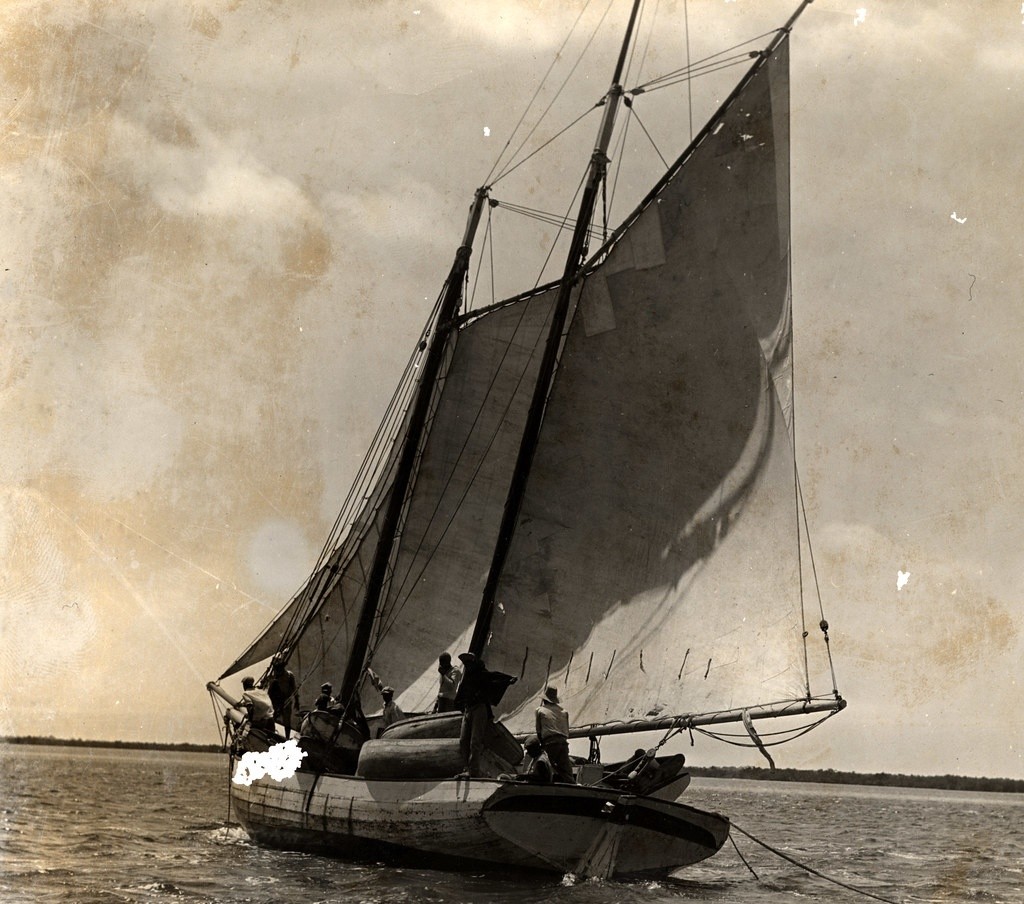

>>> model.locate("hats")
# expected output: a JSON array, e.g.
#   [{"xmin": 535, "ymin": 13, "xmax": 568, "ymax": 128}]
[
  {"xmin": 380, "ymin": 686, "xmax": 394, "ymax": 695},
  {"xmin": 524, "ymin": 736, "xmax": 539, "ymax": 749},
  {"xmin": 272, "ymin": 658, "xmax": 288, "ymax": 665},
  {"xmin": 459, "ymin": 654, "xmax": 485, "ymax": 672},
  {"xmin": 539, "ymin": 686, "xmax": 559, "ymax": 704},
  {"xmin": 241, "ymin": 677, "xmax": 255, "ymax": 682}
]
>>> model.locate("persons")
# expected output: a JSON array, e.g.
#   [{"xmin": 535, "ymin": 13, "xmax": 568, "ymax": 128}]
[
  {"xmin": 235, "ymin": 678, "xmax": 276, "ymax": 734},
  {"xmin": 453, "ymin": 651, "xmax": 519, "ymax": 781},
  {"xmin": 314, "ymin": 681, "xmax": 359, "ymax": 733},
  {"xmin": 381, "ymin": 685, "xmax": 407, "ymax": 728},
  {"xmin": 431, "ymin": 652, "xmax": 459, "ymax": 713},
  {"xmin": 494, "ymin": 736, "xmax": 556, "ymax": 785},
  {"xmin": 535, "ymin": 687, "xmax": 580, "ymax": 783},
  {"xmin": 268, "ymin": 661, "xmax": 298, "ymax": 740}
]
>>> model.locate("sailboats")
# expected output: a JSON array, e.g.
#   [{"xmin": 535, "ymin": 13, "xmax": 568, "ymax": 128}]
[{"xmin": 205, "ymin": 0, "xmax": 848, "ymax": 879}]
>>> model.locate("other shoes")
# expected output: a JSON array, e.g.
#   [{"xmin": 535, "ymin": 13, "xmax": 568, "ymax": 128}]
[{"xmin": 454, "ymin": 770, "xmax": 475, "ymax": 780}]
